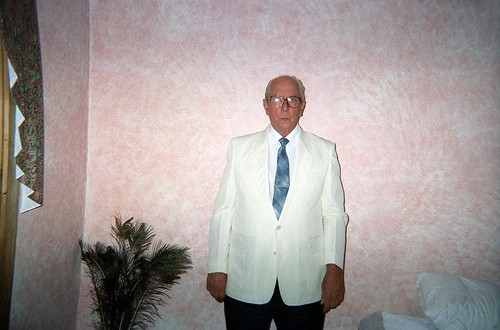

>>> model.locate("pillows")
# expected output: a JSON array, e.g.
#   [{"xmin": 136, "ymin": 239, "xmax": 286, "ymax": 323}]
[{"xmin": 417, "ymin": 271, "xmax": 500, "ymax": 330}]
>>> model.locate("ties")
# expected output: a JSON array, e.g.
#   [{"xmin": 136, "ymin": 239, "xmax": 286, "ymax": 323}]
[{"xmin": 272, "ymin": 138, "xmax": 290, "ymax": 221}]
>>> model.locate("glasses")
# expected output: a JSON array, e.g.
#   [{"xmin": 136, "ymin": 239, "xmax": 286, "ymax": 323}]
[{"xmin": 266, "ymin": 95, "xmax": 303, "ymax": 108}]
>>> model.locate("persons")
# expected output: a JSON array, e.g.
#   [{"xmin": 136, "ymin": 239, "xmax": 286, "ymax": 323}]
[{"xmin": 206, "ymin": 75, "xmax": 349, "ymax": 330}]
